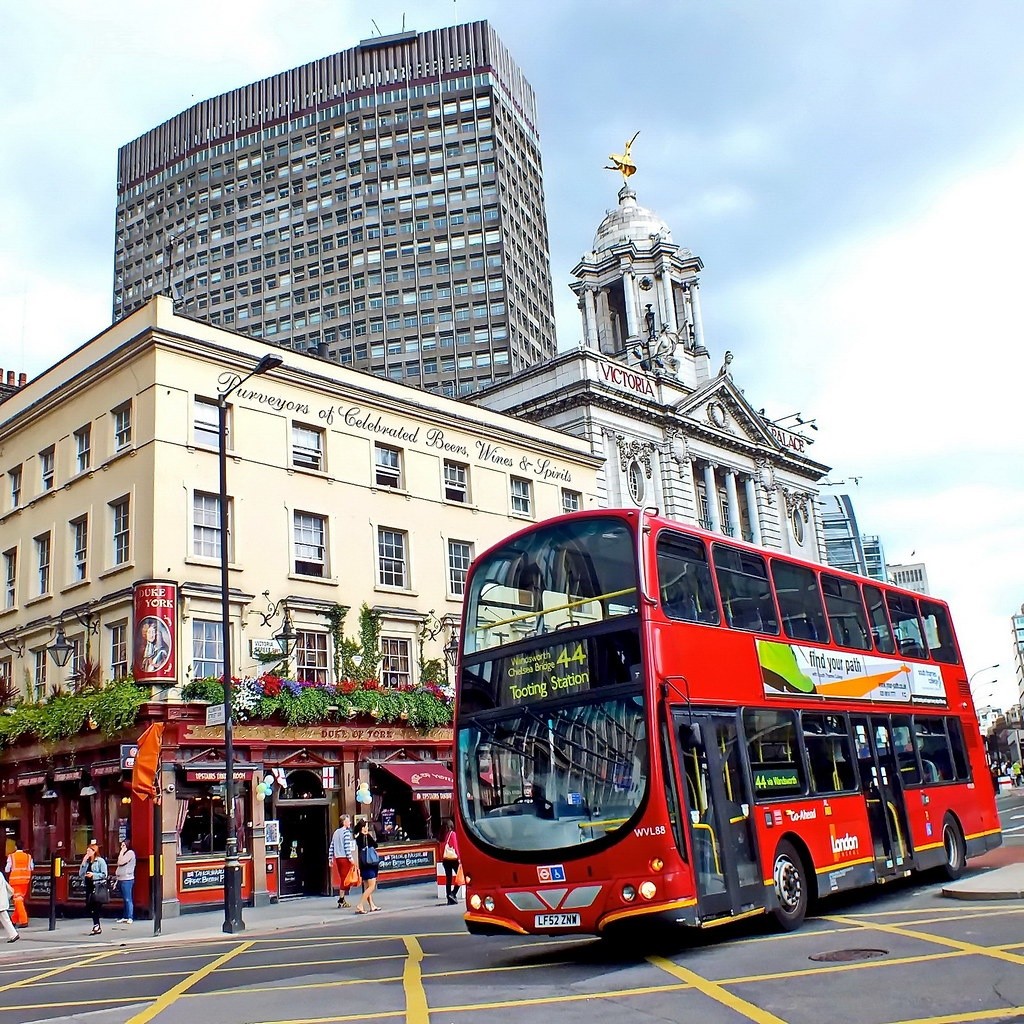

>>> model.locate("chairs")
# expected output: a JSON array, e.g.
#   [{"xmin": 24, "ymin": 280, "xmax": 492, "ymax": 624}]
[
  {"xmin": 882, "ymin": 635, "xmax": 902, "ymax": 653},
  {"xmin": 900, "ymin": 760, "xmax": 939, "ymax": 783},
  {"xmin": 697, "ymin": 608, "xmax": 715, "ymax": 624},
  {"xmin": 901, "ymin": 638, "xmax": 925, "ymax": 657},
  {"xmin": 734, "ymin": 609, "xmax": 763, "ymax": 631},
  {"xmin": 663, "ymin": 595, "xmax": 698, "ymax": 620},
  {"xmin": 711, "ymin": 605, "xmax": 731, "ymax": 627},
  {"xmin": 830, "ymin": 628, "xmax": 850, "ymax": 645},
  {"xmin": 791, "ymin": 617, "xmax": 819, "ymax": 640},
  {"xmin": 758, "ymin": 613, "xmax": 769, "ymax": 631},
  {"xmin": 774, "ymin": 613, "xmax": 794, "ymax": 636},
  {"xmin": 862, "ymin": 631, "xmax": 882, "ymax": 651}
]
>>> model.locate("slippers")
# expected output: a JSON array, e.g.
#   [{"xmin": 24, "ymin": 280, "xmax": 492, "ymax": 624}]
[
  {"xmin": 355, "ymin": 910, "xmax": 366, "ymax": 914},
  {"xmin": 370, "ymin": 907, "xmax": 381, "ymax": 912}
]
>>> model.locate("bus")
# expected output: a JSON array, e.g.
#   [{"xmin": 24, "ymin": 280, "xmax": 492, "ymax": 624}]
[{"xmin": 443, "ymin": 505, "xmax": 1003, "ymax": 949}]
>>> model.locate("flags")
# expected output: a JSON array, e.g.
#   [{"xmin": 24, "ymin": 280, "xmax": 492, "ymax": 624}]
[
  {"xmin": 272, "ymin": 767, "xmax": 287, "ymax": 788},
  {"xmin": 322, "ymin": 767, "xmax": 335, "ymax": 788}
]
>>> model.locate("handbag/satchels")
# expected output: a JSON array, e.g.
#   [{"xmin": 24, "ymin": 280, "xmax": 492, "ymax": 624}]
[
  {"xmin": 443, "ymin": 830, "xmax": 458, "ymax": 860},
  {"xmin": 93, "ymin": 872, "xmax": 110, "ymax": 905},
  {"xmin": 344, "ymin": 865, "xmax": 361, "ymax": 887},
  {"xmin": 455, "ymin": 864, "xmax": 465, "ymax": 886},
  {"xmin": 361, "ymin": 833, "xmax": 379, "ymax": 865}
]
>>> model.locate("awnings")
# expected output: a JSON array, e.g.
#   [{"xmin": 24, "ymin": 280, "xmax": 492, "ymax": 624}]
[
  {"xmin": 480, "ymin": 762, "xmax": 532, "ymax": 795},
  {"xmin": 379, "ymin": 761, "xmax": 471, "ymax": 801}
]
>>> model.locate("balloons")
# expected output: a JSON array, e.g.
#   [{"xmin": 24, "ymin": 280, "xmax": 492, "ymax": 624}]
[
  {"xmin": 256, "ymin": 774, "xmax": 274, "ymax": 801},
  {"xmin": 356, "ymin": 783, "xmax": 372, "ymax": 805}
]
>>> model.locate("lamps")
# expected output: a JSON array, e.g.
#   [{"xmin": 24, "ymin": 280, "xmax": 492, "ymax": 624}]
[
  {"xmin": 970, "ymin": 676, "xmax": 1003, "ymax": 694},
  {"xmin": 46, "ymin": 607, "xmax": 99, "ymax": 665},
  {"xmin": 964, "ymin": 660, "xmax": 1001, "ymax": 684},
  {"xmin": 972, "ymin": 690, "xmax": 995, "ymax": 700},
  {"xmin": 786, "ymin": 418, "xmax": 819, "ymax": 430},
  {"xmin": 773, "ymin": 411, "xmax": 803, "ymax": 426},
  {"xmin": 631, "ymin": 352, "xmax": 668, "ymax": 368},
  {"xmin": 975, "ymin": 703, "xmax": 994, "ymax": 712},
  {"xmin": 608, "ymin": 344, "xmax": 642, "ymax": 361},
  {"xmin": 259, "ymin": 589, "xmax": 301, "ymax": 658},
  {"xmin": 427, "ymin": 609, "xmax": 459, "ymax": 667}
]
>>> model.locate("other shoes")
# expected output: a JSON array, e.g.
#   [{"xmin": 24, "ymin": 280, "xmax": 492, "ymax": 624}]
[
  {"xmin": 339, "ymin": 899, "xmax": 351, "ymax": 908},
  {"xmin": 17, "ymin": 923, "xmax": 28, "ymax": 928},
  {"xmin": 91, "ymin": 924, "xmax": 102, "ymax": 934},
  {"xmin": 117, "ymin": 918, "xmax": 128, "ymax": 923},
  {"xmin": 7, "ymin": 935, "xmax": 19, "ymax": 943},
  {"xmin": 127, "ymin": 917, "xmax": 133, "ymax": 924},
  {"xmin": 447, "ymin": 900, "xmax": 455, "ymax": 904},
  {"xmin": 449, "ymin": 893, "xmax": 458, "ymax": 904}
]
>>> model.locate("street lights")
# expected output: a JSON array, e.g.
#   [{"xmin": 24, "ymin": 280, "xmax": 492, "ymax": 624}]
[{"xmin": 221, "ymin": 352, "xmax": 285, "ymax": 934}]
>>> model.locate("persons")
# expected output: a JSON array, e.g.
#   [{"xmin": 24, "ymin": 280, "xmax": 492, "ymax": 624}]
[
  {"xmin": 0, "ymin": 871, "xmax": 20, "ymax": 943},
  {"xmin": 115, "ymin": 840, "xmax": 136, "ymax": 925},
  {"xmin": 604, "ymin": 130, "xmax": 640, "ymax": 185},
  {"xmin": 354, "ymin": 819, "xmax": 382, "ymax": 915},
  {"xmin": 436, "ymin": 819, "xmax": 462, "ymax": 904},
  {"xmin": 989, "ymin": 759, "xmax": 1024, "ymax": 794},
  {"xmin": 328, "ymin": 813, "xmax": 354, "ymax": 907},
  {"xmin": 5, "ymin": 839, "xmax": 35, "ymax": 928},
  {"xmin": 719, "ymin": 349, "xmax": 734, "ymax": 379},
  {"xmin": 654, "ymin": 322, "xmax": 679, "ymax": 377},
  {"xmin": 79, "ymin": 844, "xmax": 107, "ymax": 935}
]
